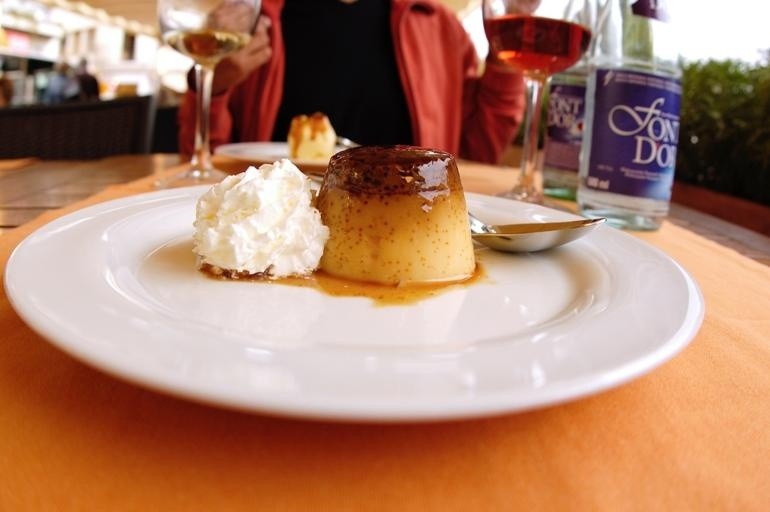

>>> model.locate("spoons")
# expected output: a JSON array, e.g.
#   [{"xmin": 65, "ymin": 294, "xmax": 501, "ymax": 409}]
[{"xmin": 298, "ymin": 168, "xmax": 608, "ymax": 260}]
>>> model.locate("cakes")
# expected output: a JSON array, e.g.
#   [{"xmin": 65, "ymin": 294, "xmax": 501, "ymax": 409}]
[
  {"xmin": 316, "ymin": 144, "xmax": 476, "ymax": 286},
  {"xmin": 195, "ymin": 158, "xmax": 329, "ymax": 281},
  {"xmin": 287, "ymin": 111, "xmax": 337, "ymax": 164}
]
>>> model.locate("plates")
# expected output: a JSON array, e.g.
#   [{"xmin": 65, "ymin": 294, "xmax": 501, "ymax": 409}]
[
  {"xmin": 2, "ymin": 178, "xmax": 705, "ymax": 428},
  {"xmin": 214, "ymin": 140, "xmax": 350, "ymax": 166}
]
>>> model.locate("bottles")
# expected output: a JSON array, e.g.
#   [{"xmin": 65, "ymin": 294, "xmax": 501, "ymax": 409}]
[
  {"xmin": 579, "ymin": 0, "xmax": 685, "ymax": 232},
  {"xmin": 544, "ymin": 0, "xmax": 620, "ymax": 201}
]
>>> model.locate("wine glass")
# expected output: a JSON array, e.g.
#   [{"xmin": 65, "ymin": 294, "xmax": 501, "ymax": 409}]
[
  {"xmin": 481, "ymin": 0, "xmax": 590, "ymax": 204},
  {"xmin": 152, "ymin": 0, "xmax": 263, "ymax": 192}
]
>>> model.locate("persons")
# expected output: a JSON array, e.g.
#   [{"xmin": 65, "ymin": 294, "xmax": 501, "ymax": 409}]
[
  {"xmin": 175, "ymin": 0, "xmax": 531, "ymax": 165},
  {"xmin": 75, "ymin": 58, "xmax": 99, "ymax": 102},
  {"xmin": 42, "ymin": 58, "xmax": 72, "ymax": 107}
]
